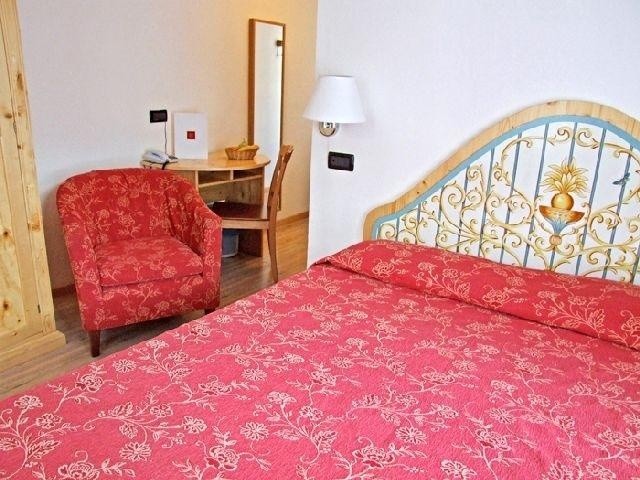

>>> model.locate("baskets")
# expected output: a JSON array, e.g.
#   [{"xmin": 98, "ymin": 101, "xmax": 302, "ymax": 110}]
[{"xmin": 224, "ymin": 144, "xmax": 260, "ymax": 160}]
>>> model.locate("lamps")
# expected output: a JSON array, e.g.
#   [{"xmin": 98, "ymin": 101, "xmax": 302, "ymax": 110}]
[{"xmin": 302, "ymin": 74, "xmax": 367, "ymax": 136}]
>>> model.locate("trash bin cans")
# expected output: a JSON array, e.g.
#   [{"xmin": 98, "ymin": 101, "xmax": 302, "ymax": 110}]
[{"xmin": 222, "ymin": 230, "xmax": 239, "ymax": 258}]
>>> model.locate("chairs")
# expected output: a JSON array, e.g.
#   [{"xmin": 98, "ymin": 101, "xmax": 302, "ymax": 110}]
[
  {"xmin": 212, "ymin": 144, "xmax": 295, "ymax": 283},
  {"xmin": 56, "ymin": 168, "xmax": 224, "ymax": 357}
]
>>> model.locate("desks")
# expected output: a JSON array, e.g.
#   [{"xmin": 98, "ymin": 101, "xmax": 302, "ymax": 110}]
[{"xmin": 140, "ymin": 149, "xmax": 271, "ymax": 258}]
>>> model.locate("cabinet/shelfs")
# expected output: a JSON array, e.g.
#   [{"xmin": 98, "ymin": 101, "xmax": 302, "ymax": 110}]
[{"xmin": 0, "ymin": 0, "xmax": 66, "ymax": 395}]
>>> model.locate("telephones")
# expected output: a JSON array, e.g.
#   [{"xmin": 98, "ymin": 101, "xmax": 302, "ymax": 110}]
[{"xmin": 143, "ymin": 150, "xmax": 171, "ymax": 164}]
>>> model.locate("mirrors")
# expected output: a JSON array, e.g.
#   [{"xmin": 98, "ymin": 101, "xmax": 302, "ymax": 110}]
[{"xmin": 248, "ymin": 17, "xmax": 286, "ymax": 213}]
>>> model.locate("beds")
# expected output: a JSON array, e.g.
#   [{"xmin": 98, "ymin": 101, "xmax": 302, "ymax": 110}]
[{"xmin": 0, "ymin": 99, "xmax": 638, "ymax": 480}]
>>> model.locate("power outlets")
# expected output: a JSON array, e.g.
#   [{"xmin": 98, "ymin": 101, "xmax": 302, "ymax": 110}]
[
  {"xmin": 328, "ymin": 152, "xmax": 354, "ymax": 171},
  {"xmin": 150, "ymin": 109, "xmax": 167, "ymax": 122}
]
